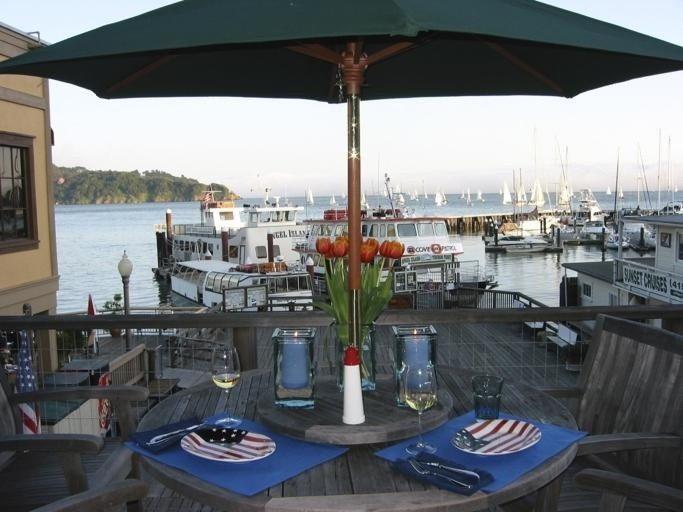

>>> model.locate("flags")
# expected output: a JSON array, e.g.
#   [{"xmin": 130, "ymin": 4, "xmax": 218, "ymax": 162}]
[
  {"xmin": 14, "ymin": 320, "xmax": 41, "ymax": 434},
  {"xmin": 82, "ymin": 293, "xmax": 100, "ymax": 362}
]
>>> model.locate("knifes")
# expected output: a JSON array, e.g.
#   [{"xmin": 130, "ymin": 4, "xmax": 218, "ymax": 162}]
[{"xmin": 417, "ymin": 458, "xmax": 482, "ymax": 481}]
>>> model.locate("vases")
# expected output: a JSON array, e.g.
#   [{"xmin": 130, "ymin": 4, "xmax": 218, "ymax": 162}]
[{"xmin": 329, "ymin": 321, "xmax": 377, "ymax": 391}]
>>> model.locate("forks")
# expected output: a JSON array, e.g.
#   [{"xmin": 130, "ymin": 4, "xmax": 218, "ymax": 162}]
[{"xmin": 406, "ymin": 457, "xmax": 473, "ymax": 492}]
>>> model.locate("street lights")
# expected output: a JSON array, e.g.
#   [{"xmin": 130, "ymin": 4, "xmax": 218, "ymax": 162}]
[{"xmin": 118, "ymin": 250, "xmax": 134, "ymax": 352}]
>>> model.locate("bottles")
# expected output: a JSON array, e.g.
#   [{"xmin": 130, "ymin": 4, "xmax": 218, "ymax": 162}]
[{"xmin": 339, "ymin": 344, "xmax": 368, "ymax": 426}]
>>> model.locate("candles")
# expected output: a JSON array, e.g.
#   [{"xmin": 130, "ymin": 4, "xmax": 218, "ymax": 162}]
[
  {"xmin": 404, "ymin": 329, "xmax": 428, "ymax": 390},
  {"xmin": 282, "ymin": 332, "xmax": 310, "ymax": 387}
]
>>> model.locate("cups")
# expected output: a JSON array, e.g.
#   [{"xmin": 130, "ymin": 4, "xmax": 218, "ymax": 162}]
[{"xmin": 471, "ymin": 374, "xmax": 506, "ymax": 421}]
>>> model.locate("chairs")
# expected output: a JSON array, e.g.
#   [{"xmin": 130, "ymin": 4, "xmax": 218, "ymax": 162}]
[
  {"xmin": 0, "ymin": 373, "xmax": 151, "ymax": 512},
  {"xmin": 574, "ymin": 467, "xmax": 682, "ymax": 511},
  {"xmin": 537, "ymin": 312, "xmax": 681, "ymax": 512},
  {"xmin": 33, "ymin": 476, "xmax": 147, "ymax": 510}
]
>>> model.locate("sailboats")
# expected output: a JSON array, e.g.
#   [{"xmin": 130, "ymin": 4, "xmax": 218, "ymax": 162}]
[
  {"xmin": 484, "ymin": 133, "xmax": 682, "ymax": 254},
  {"xmin": 302, "ymin": 181, "xmax": 485, "ymax": 211}
]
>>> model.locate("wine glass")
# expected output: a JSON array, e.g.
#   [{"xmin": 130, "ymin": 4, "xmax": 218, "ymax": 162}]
[
  {"xmin": 208, "ymin": 344, "xmax": 241, "ymax": 429},
  {"xmin": 402, "ymin": 365, "xmax": 441, "ymax": 455}
]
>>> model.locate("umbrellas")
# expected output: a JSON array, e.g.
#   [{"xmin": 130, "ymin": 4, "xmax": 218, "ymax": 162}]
[{"xmin": 0, "ymin": 0, "xmax": 683, "ymax": 346}]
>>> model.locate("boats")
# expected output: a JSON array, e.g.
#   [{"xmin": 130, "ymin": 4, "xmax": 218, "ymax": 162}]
[
  {"xmin": 291, "ymin": 172, "xmax": 498, "ymax": 309},
  {"xmin": 152, "ymin": 183, "xmax": 317, "ymax": 312}
]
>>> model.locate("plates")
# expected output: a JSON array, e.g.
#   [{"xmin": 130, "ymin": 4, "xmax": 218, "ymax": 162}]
[{"xmin": 451, "ymin": 418, "xmax": 541, "ymax": 456}]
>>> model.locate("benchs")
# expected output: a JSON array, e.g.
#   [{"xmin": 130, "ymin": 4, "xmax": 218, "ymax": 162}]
[
  {"xmin": 524, "ymin": 320, "xmax": 544, "ymax": 341},
  {"xmin": 544, "ymin": 325, "xmax": 577, "ymax": 359}
]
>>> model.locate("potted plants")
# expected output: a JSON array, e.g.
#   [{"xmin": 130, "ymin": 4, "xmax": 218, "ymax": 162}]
[{"xmin": 100, "ymin": 293, "xmax": 126, "ymax": 337}]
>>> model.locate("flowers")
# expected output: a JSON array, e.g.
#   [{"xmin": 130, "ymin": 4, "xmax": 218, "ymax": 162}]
[{"xmin": 312, "ymin": 232, "xmax": 405, "ymax": 365}]
